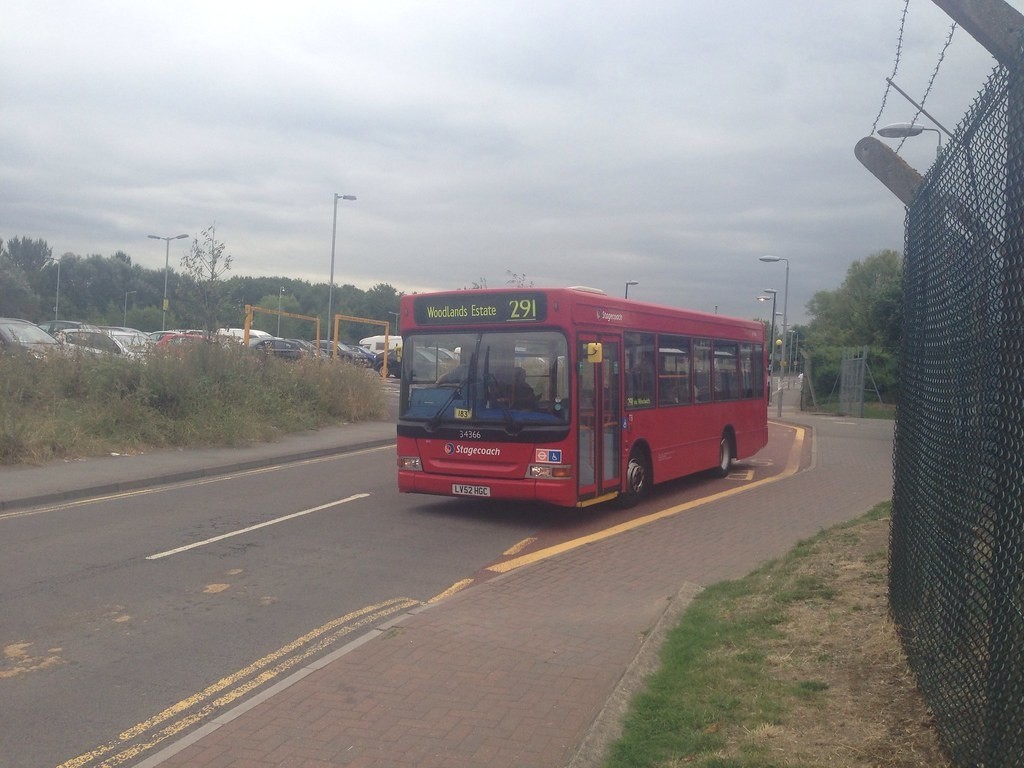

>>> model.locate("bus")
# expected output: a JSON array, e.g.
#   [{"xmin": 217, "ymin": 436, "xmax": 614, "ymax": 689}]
[{"xmin": 395, "ymin": 285, "xmax": 769, "ymax": 510}]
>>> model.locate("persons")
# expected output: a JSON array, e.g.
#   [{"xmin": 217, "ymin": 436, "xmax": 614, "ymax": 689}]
[{"xmin": 515, "ymin": 367, "xmax": 542, "ymax": 406}]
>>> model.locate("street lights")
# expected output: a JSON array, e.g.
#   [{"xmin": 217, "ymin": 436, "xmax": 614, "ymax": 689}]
[
  {"xmin": 388, "ymin": 311, "xmax": 401, "ymax": 336},
  {"xmin": 786, "ymin": 328, "xmax": 799, "ymax": 388},
  {"xmin": 781, "ymin": 322, "xmax": 793, "ymax": 390},
  {"xmin": 876, "ymin": 117, "xmax": 946, "ymax": 251},
  {"xmin": 623, "ymin": 281, "xmax": 638, "ymax": 300},
  {"xmin": 147, "ymin": 233, "xmax": 190, "ymax": 331},
  {"xmin": 756, "ymin": 295, "xmax": 775, "ymax": 406},
  {"xmin": 123, "ymin": 291, "xmax": 137, "ymax": 328},
  {"xmin": 327, "ymin": 194, "xmax": 358, "ymax": 356},
  {"xmin": 45, "ymin": 256, "xmax": 60, "ymax": 320},
  {"xmin": 758, "ymin": 255, "xmax": 789, "ymax": 417},
  {"xmin": 764, "ymin": 288, "xmax": 778, "ymax": 402},
  {"xmin": 276, "ymin": 287, "xmax": 287, "ymax": 338},
  {"xmin": 624, "ymin": 280, "xmax": 638, "ymax": 300}
]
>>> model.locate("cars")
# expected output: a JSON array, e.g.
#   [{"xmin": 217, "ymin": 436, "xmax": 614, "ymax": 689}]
[
  {"xmin": 0, "ymin": 317, "xmax": 62, "ymax": 358},
  {"xmin": 55, "ymin": 329, "xmax": 156, "ymax": 364},
  {"xmin": 34, "ymin": 319, "xmax": 460, "ymax": 381}
]
